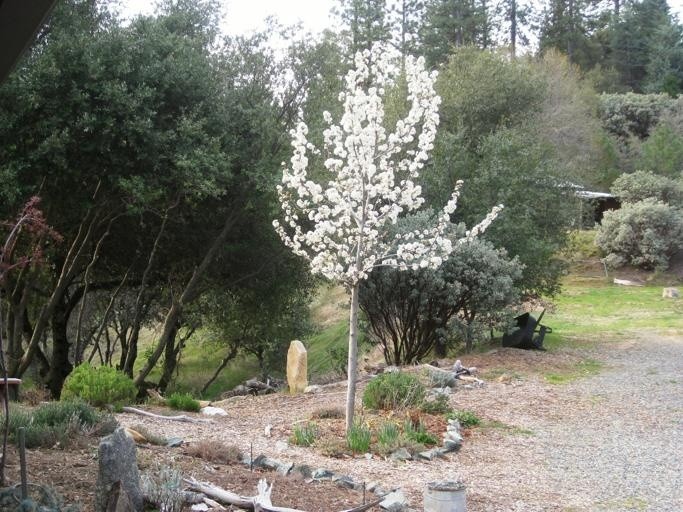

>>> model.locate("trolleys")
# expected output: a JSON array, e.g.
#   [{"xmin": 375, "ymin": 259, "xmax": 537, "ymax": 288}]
[{"xmin": 502, "ymin": 308, "xmax": 553, "ymax": 351}]
